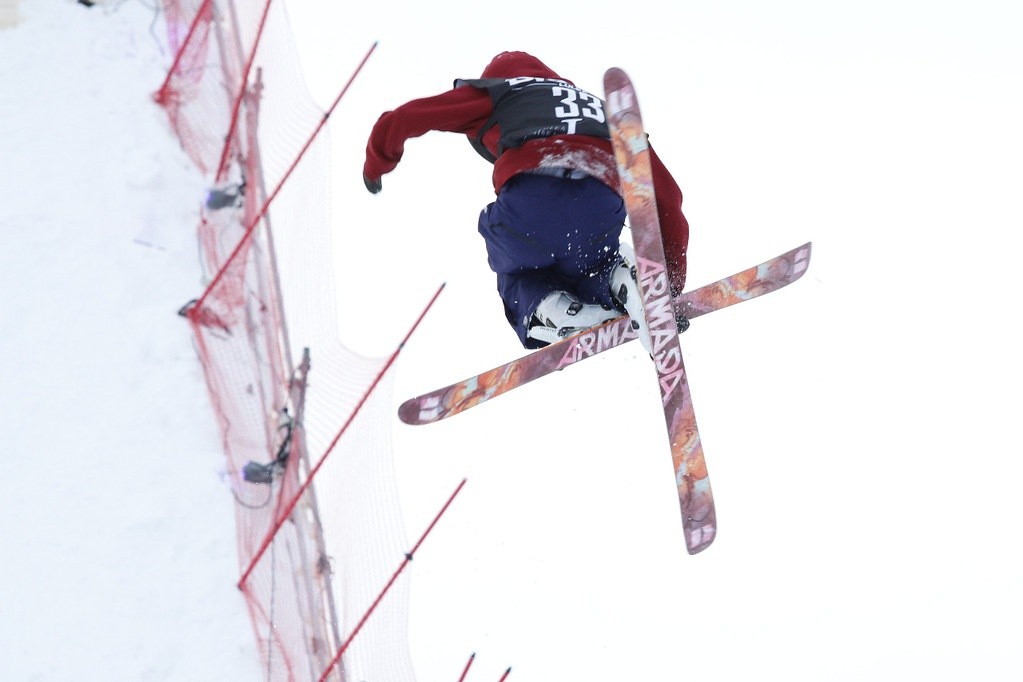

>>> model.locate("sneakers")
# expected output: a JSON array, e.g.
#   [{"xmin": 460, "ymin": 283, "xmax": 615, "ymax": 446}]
[
  {"xmin": 535, "ymin": 291, "xmax": 623, "ymax": 338},
  {"xmin": 611, "ymin": 265, "xmax": 654, "ymax": 360}
]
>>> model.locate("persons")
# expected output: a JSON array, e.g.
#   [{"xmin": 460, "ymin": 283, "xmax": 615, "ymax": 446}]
[{"xmin": 361, "ymin": 49, "xmax": 690, "ymax": 350}]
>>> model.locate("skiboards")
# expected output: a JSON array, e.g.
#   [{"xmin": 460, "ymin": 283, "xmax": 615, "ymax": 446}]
[{"xmin": 397, "ymin": 65, "xmax": 813, "ymax": 555}]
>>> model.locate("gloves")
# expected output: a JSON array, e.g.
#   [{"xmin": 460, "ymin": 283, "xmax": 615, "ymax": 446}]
[
  {"xmin": 675, "ymin": 316, "xmax": 690, "ymax": 334},
  {"xmin": 363, "ymin": 171, "xmax": 382, "ymax": 194}
]
